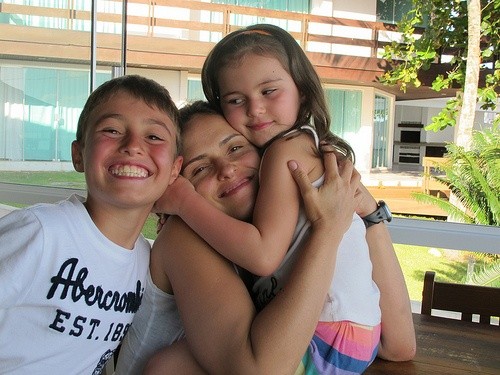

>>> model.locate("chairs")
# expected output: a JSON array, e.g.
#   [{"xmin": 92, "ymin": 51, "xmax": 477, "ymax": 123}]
[{"xmin": 421, "ymin": 271, "xmax": 500, "ymax": 325}]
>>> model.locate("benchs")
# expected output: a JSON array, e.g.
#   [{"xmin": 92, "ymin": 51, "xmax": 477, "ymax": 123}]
[{"xmin": 423, "ymin": 157, "xmax": 451, "ymax": 198}]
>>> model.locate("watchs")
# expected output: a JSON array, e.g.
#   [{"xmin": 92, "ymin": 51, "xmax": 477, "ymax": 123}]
[{"xmin": 361, "ymin": 199, "xmax": 391, "ymax": 229}]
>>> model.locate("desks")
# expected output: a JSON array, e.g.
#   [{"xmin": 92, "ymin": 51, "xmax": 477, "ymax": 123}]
[{"xmin": 362, "ymin": 313, "xmax": 500, "ymax": 375}]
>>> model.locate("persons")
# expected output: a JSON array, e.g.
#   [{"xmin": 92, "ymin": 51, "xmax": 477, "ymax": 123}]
[
  {"xmin": 149, "ymin": 23, "xmax": 383, "ymax": 375},
  {"xmin": 111, "ymin": 101, "xmax": 416, "ymax": 375},
  {"xmin": 2, "ymin": 74, "xmax": 184, "ymax": 374}
]
